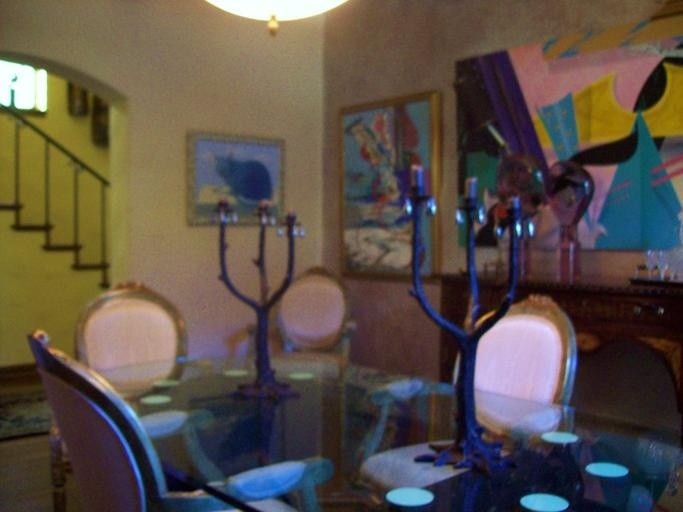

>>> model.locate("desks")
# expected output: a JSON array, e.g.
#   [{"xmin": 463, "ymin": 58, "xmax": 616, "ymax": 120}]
[
  {"xmin": 47, "ymin": 354, "xmax": 683, "ymax": 512},
  {"xmin": 433, "ymin": 270, "xmax": 683, "ymax": 433}
]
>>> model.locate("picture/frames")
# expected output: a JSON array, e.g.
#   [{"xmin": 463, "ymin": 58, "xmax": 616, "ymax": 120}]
[
  {"xmin": 187, "ymin": 130, "xmax": 287, "ymax": 226},
  {"xmin": 338, "ymin": 89, "xmax": 444, "ymax": 284}
]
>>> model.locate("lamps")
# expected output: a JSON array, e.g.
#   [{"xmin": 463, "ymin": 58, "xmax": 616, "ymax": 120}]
[
  {"xmin": 204, "ymin": 0, "xmax": 348, "ymax": 36},
  {"xmin": 403, "ymin": 166, "xmax": 534, "ymax": 469},
  {"xmin": 219, "ymin": 201, "xmax": 305, "ymax": 399}
]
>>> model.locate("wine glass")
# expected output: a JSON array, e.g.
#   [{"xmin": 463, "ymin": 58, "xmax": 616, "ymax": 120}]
[{"xmin": 642, "ymin": 248, "xmax": 669, "ymax": 281}]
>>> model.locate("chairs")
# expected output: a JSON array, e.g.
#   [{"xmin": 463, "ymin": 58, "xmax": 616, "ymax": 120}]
[
  {"xmin": 26, "ymin": 328, "xmax": 301, "ymax": 512},
  {"xmin": 275, "ymin": 265, "xmax": 355, "ymax": 359},
  {"xmin": 73, "ymin": 278, "xmax": 189, "ymax": 399},
  {"xmin": 358, "ymin": 293, "xmax": 580, "ymax": 500}
]
